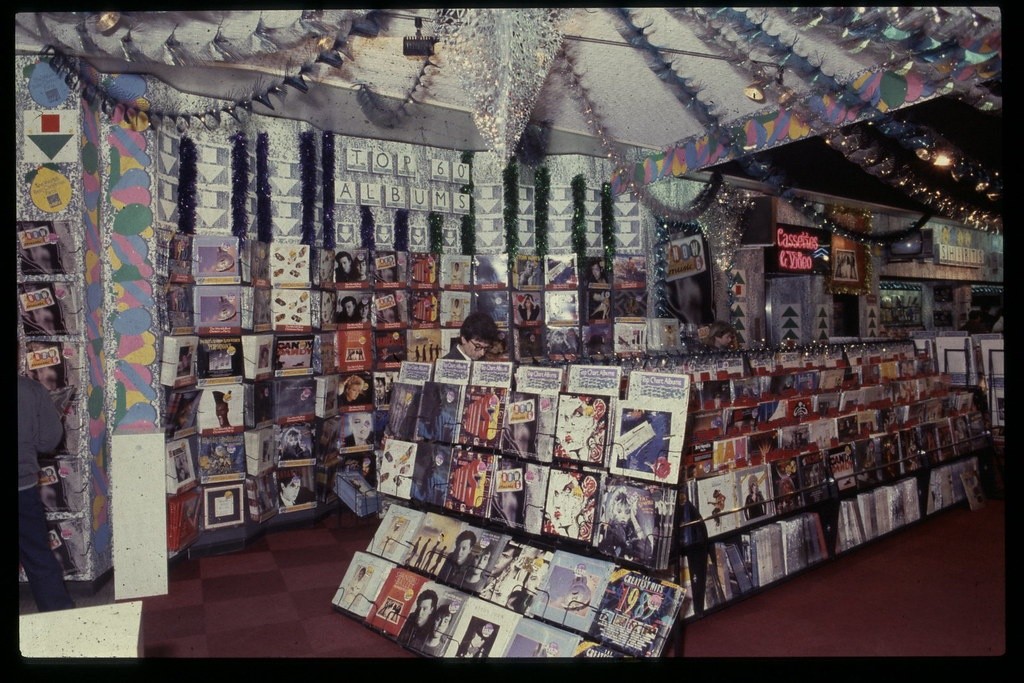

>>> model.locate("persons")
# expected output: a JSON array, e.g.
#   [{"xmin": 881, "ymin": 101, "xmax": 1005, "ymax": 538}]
[
  {"xmin": 278, "ymin": 473, "xmax": 300, "ymax": 508},
  {"xmin": 398, "ymin": 589, "xmax": 438, "ymax": 650},
  {"xmin": 335, "ymin": 251, "xmax": 354, "ymax": 282},
  {"xmin": 507, "ymin": 560, "xmax": 550, "ymax": 616},
  {"xmin": 480, "ymin": 544, "xmax": 521, "ymax": 607},
  {"xmin": 600, "ymin": 487, "xmax": 651, "ymax": 565},
  {"xmin": 336, "ymin": 295, "xmax": 361, "ymax": 324},
  {"xmin": 348, "ymin": 255, "xmax": 369, "ymax": 282},
  {"xmin": 345, "ymin": 412, "xmax": 374, "ymax": 446},
  {"xmin": 438, "ymin": 531, "xmax": 476, "ymax": 590},
  {"xmin": 410, "ymin": 604, "xmax": 452, "ymax": 656},
  {"xmin": 744, "ymin": 482, "xmax": 766, "ymax": 519},
  {"xmin": 518, "ymin": 293, "xmax": 540, "ymax": 322},
  {"xmin": 17, "ymin": 375, "xmax": 74, "ymax": 614},
  {"xmin": 708, "ymin": 321, "xmax": 734, "ymax": 352},
  {"xmin": 337, "ymin": 376, "xmax": 364, "ymax": 408},
  {"xmin": 358, "ymin": 297, "xmax": 371, "ymax": 323},
  {"xmin": 965, "ymin": 299, "xmax": 1003, "ymax": 334},
  {"xmin": 441, "ymin": 312, "xmax": 499, "ymax": 364}
]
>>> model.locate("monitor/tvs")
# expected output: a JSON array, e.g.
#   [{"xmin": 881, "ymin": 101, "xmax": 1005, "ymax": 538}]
[{"xmin": 884, "ymin": 228, "xmax": 936, "ymax": 261}]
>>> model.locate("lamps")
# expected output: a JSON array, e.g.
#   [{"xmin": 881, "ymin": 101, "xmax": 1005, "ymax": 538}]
[
  {"xmin": 95, "ymin": 12, "xmax": 120, "ymax": 32},
  {"xmin": 402, "ymin": 17, "xmax": 437, "ymax": 57},
  {"xmin": 744, "ymin": 74, "xmax": 768, "ymax": 100},
  {"xmin": 916, "ymin": 147, "xmax": 933, "ymax": 161}
]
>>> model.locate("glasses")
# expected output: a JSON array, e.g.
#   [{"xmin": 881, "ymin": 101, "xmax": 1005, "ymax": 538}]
[{"xmin": 470, "ymin": 339, "xmax": 491, "ymax": 352}]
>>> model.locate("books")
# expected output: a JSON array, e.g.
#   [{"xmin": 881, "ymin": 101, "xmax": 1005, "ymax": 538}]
[
  {"xmin": 155, "ymin": 236, "xmax": 648, "ymax": 523},
  {"xmin": 342, "ymin": 356, "xmax": 989, "ymax": 658},
  {"xmin": 17, "ymin": 216, "xmax": 84, "ymax": 579}
]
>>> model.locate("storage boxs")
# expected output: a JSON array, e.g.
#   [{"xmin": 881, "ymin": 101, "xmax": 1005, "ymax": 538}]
[
  {"xmin": 245, "ymin": 474, "xmax": 278, "ymax": 524},
  {"xmin": 253, "ymin": 288, "xmax": 312, "ymax": 334},
  {"xmin": 314, "ymin": 332, "xmax": 336, "ymax": 376},
  {"xmin": 314, "ymin": 376, "xmax": 338, "ymax": 419},
  {"xmin": 197, "ymin": 435, "xmax": 243, "ymax": 485},
  {"xmin": 191, "ymin": 285, "xmax": 241, "ymax": 337},
  {"xmin": 195, "ymin": 383, "xmax": 244, "ymax": 434},
  {"xmin": 202, "ymin": 483, "xmax": 244, "ymax": 529},
  {"xmin": 243, "ymin": 334, "xmax": 273, "ymax": 379},
  {"xmin": 245, "ymin": 429, "xmax": 274, "ymax": 475},
  {"xmin": 242, "ymin": 382, "xmax": 274, "ymax": 429},
  {"xmin": 161, "ymin": 335, "xmax": 199, "ymax": 388},
  {"xmin": 198, "ymin": 339, "xmax": 243, "ymax": 387},
  {"xmin": 311, "ymin": 290, "xmax": 335, "ymax": 331},
  {"xmin": 255, "ymin": 242, "xmax": 310, "ymax": 288},
  {"xmin": 190, "ymin": 235, "xmax": 240, "ymax": 283},
  {"xmin": 311, "ymin": 248, "xmax": 336, "ymax": 289}
]
